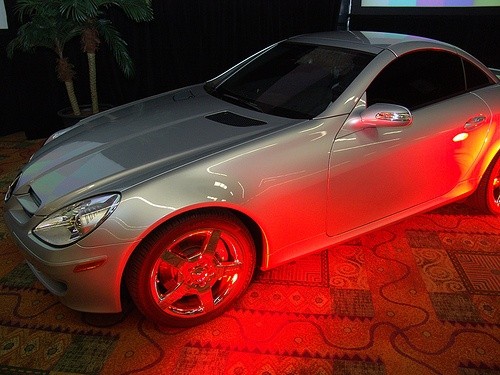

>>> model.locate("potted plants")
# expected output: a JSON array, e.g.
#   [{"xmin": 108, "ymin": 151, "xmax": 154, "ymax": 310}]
[{"xmin": 5, "ymin": 0, "xmax": 154, "ymax": 129}]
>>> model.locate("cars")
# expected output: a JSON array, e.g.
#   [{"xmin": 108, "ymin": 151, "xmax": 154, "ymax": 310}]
[{"xmin": 0, "ymin": 31, "xmax": 500, "ymax": 330}]
